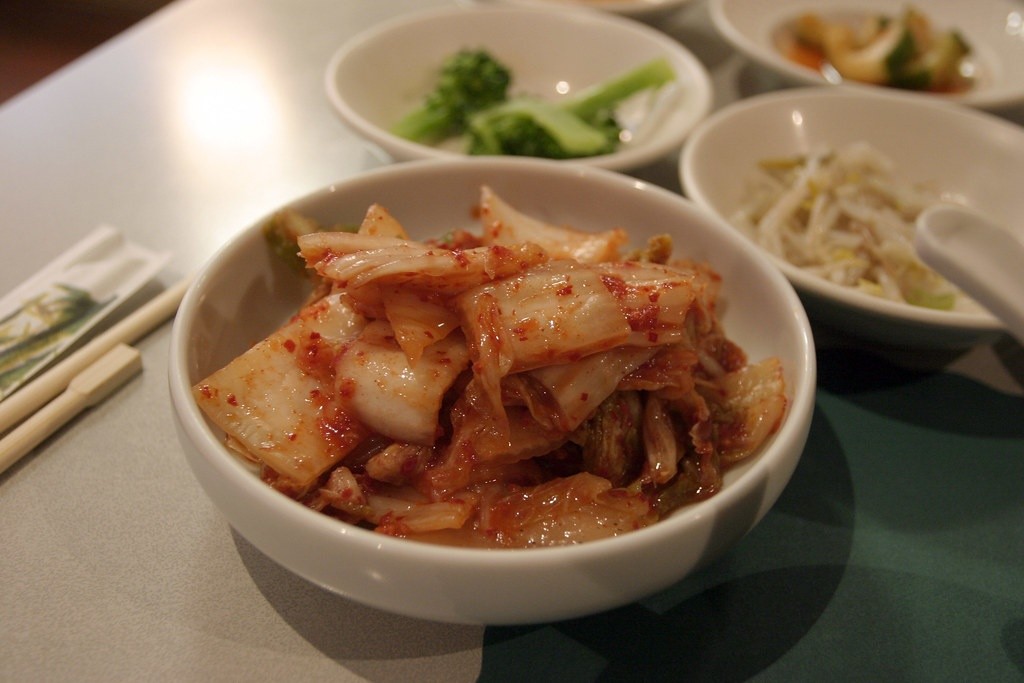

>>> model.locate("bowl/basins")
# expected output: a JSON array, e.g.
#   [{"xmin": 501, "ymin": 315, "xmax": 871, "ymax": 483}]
[
  {"xmin": 704, "ymin": 0, "xmax": 1023, "ymax": 110},
  {"xmin": 167, "ymin": 155, "xmax": 818, "ymax": 627},
  {"xmin": 320, "ymin": 0, "xmax": 713, "ymax": 174},
  {"xmin": 677, "ymin": 84, "xmax": 1024, "ymax": 352}
]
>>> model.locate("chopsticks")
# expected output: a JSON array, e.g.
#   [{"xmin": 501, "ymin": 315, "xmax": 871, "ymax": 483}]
[{"xmin": 0, "ymin": 272, "xmax": 194, "ymax": 475}]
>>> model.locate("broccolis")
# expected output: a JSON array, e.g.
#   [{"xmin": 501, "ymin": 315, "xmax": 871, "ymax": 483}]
[{"xmin": 393, "ymin": 44, "xmax": 675, "ymax": 158}]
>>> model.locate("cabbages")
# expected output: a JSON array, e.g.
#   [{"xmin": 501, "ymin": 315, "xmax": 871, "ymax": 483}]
[{"xmin": 192, "ymin": 186, "xmax": 785, "ymax": 552}]
